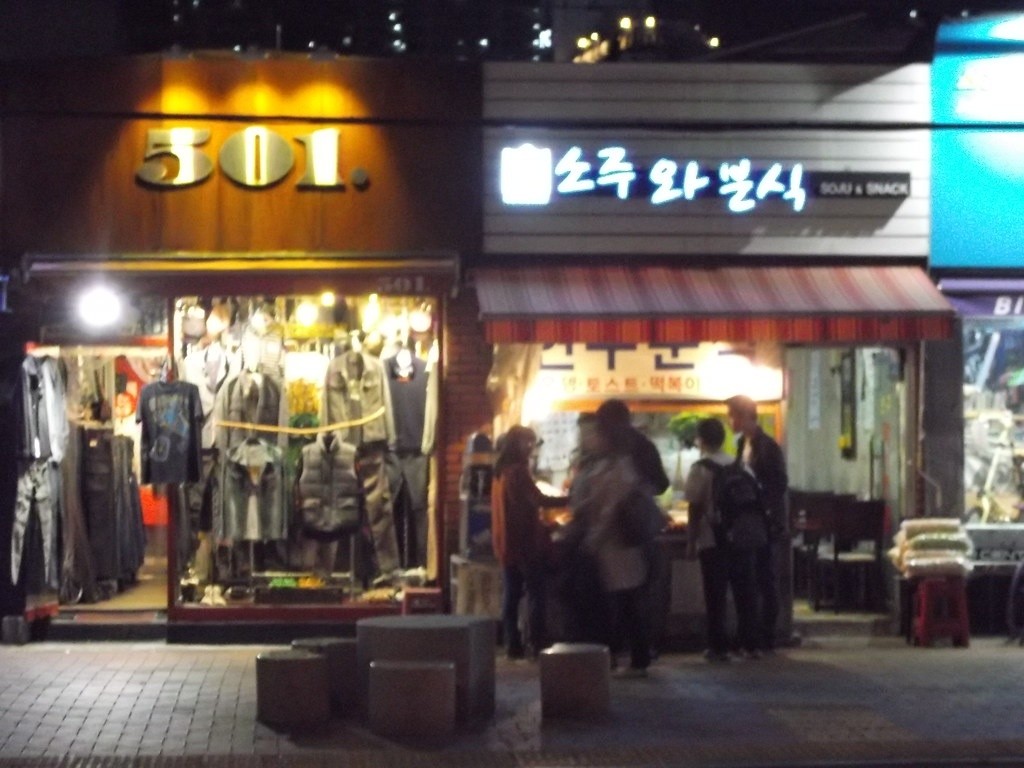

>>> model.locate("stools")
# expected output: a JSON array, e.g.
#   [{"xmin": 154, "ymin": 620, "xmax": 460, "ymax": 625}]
[
  {"xmin": 539, "ymin": 643, "xmax": 611, "ymax": 728},
  {"xmin": 911, "ymin": 577, "xmax": 970, "ymax": 648},
  {"xmin": 256, "ymin": 650, "xmax": 332, "ymax": 727},
  {"xmin": 292, "ymin": 636, "xmax": 356, "ymax": 712},
  {"xmin": 369, "ymin": 660, "xmax": 457, "ymax": 741}
]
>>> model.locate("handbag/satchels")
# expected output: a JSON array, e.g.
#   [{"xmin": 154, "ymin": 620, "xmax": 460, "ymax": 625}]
[{"xmin": 619, "ymin": 488, "xmax": 671, "ymax": 544}]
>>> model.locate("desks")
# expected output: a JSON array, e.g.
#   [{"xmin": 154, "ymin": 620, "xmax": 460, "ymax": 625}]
[{"xmin": 357, "ymin": 614, "xmax": 495, "ymax": 720}]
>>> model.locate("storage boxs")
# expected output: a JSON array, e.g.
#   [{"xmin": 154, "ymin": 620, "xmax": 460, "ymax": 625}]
[{"xmin": 254, "ymin": 586, "xmax": 343, "ymax": 605}]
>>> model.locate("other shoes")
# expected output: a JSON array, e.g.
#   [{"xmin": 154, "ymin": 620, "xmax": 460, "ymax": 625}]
[
  {"xmin": 613, "ymin": 664, "xmax": 646, "ymax": 679},
  {"xmin": 740, "ymin": 646, "xmax": 764, "ymax": 660},
  {"xmin": 703, "ymin": 649, "xmax": 730, "ymax": 664}
]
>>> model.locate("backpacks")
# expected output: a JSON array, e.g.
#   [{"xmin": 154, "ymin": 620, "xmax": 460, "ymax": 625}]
[{"xmin": 692, "ymin": 459, "xmax": 769, "ymax": 551}]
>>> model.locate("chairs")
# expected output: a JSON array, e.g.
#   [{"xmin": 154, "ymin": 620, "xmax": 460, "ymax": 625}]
[{"xmin": 790, "ymin": 489, "xmax": 887, "ymax": 615}]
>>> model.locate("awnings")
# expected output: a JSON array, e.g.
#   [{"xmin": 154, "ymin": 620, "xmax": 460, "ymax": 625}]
[{"xmin": 474, "ymin": 263, "xmax": 954, "ymax": 344}]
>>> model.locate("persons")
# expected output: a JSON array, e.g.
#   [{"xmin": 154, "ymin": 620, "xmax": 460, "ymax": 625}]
[
  {"xmin": 723, "ymin": 395, "xmax": 788, "ymax": 653},
  {"xmin": 568, "ymin": 401, "xmax": 670, "ymax": 670},
  {"xmin": 493, "ymin": 426, "xmax": 567, "ymax": 658},
  {"xmin": 685, "ymin": 419, "xmax": 764, "ymax": 663}
]
